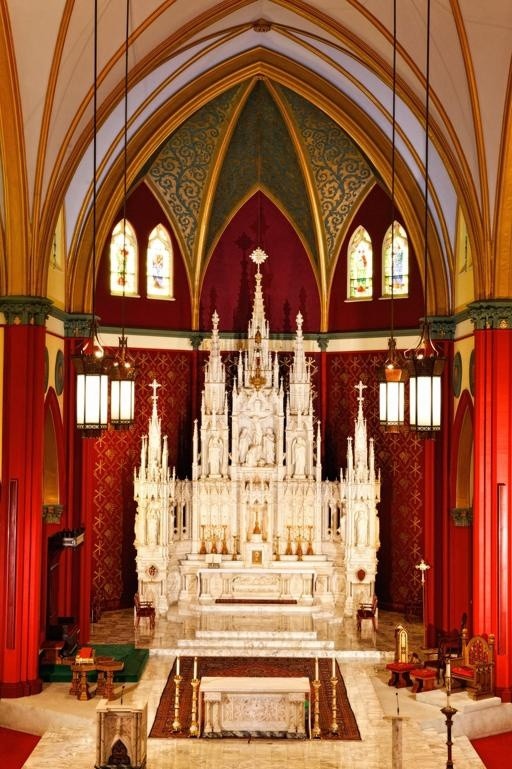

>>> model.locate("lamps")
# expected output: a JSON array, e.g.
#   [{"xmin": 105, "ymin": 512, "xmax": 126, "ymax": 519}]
[
  {"xmin": 376, "ymin": 0, "xmax": 444, "ymax": 441},
  {"xmin": 72, "ymin": 0, "xmax": 139, "ymax": 437}
]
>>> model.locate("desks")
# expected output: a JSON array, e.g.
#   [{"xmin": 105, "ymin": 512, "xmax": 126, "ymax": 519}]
[{"xmin": 199, "ymin": 676, "xmax": 312, "ymax": 740}]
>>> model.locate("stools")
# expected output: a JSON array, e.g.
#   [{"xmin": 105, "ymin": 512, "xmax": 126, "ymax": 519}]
[
  {"xmin": 385, "ymin": 661, "xmax": 436, "ymax": 694},
  {"xmin": 61, "ymin": 656, "xmax": 126, "ymax": 702}
]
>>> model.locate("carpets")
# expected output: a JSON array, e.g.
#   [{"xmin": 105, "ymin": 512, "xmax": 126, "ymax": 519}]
[{"xmin": 144, "ymin": 655, "xmax": 364, "ymax": 742}]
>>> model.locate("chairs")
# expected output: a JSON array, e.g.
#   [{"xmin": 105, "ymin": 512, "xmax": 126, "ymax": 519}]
[
  {"xmin": 356, "ymin": 594, "xmax": 381, "ymax": 635},
  {"xmin": 131, "ymin": 592, "xmax": 157, "ymax": 632},
  {"xmin": 444, "ymin": 628, "xmax": 495, "ymax": 702}
]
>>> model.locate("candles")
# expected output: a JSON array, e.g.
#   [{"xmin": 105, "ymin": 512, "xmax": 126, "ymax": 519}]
[
  {"xmin": 174, "ymin": 653, "xmax": 198, "ymax": 680},
  {"xmin": 314, "ymin": 656, "xmax": 337, "ymax": 681}
]
